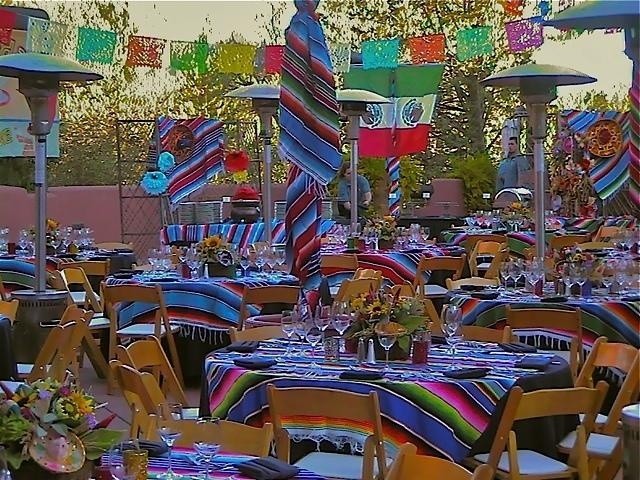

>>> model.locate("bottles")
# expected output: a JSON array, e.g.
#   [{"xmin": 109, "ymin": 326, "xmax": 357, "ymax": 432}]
[
  {"xmin": 426, "ymin": 329, "xmax": 432, "ymax": 354},
  {"xmin": 0, "ymin": 447, "xmax": 12, "ymax": 480}
]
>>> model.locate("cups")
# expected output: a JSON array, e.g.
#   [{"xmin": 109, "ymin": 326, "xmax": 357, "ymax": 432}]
[
  {"xmin": 108, "ymin": 437, "xmax": 139, "ymax": 480},
  {"xmin": 123, "ymin": 449, "xmax": 150, "ymax": 480},
  {"xmin": 413, "ymin": 330, "xmax": 429, "ymax": 366}
]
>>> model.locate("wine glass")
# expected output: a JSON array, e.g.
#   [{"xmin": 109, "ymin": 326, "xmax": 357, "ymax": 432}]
[
  {"xmin": 440, "ymin": 302, "xmax": 464, "ymax": 373},
  {"xmin": 280, "ymin": 300, "xmax": 414, "ymax": 364},
  {"xmin": 146, "ymin": 241, "xmax": 287, "ymax": 281},
  {"xmin": 463, "ymin": 206, "xmax": 563, "ymax": 238},
  {"xmin": 319, "ymin": 224, "xmax": 432, "ymax": 256},
  {"xmin": 189, "ymin": 415, "xmax": 221, "ymax": 479},
  {"xmin": 378, "ymin": 333, "xmax": 398, "ymax": 374},
  {"xmin": 153, "ymin": 402, "xmax": 185, "ymax": 480},
  {"xmin": 0, "ymin": 223, "xmax": 96, "ymax": 261},
  {"xmin": 498, "ymin": 231, "xmax": 640, "ymax": 303}
]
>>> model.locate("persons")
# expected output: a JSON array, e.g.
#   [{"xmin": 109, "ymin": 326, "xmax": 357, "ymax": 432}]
[
  {"xmin": 337, "ymin": 164, "xmax": 372, "ymax": 218},
  {"xmin": 496, "ymin": 137, "xmax": 530, "ymax": 190}
]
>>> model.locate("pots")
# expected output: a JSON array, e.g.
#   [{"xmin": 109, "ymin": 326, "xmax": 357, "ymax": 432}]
[{"xmin": 230, "ymin": 197, "xmax": 259, "ymax": 223}]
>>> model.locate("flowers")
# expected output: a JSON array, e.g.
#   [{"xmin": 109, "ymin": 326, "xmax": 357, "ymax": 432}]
[{"xmin": 503, "ymin": 203, "xmax": 531, "ymax": 222}]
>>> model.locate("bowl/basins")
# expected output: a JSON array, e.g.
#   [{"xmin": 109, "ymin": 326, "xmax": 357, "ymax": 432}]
[
  {"xmin": 120, "ymin": 269, "xmax": 145, "ymax": 275},
  {"xmin": 112, "ymin": 272, "xmax": 134, "ymax": 279}
]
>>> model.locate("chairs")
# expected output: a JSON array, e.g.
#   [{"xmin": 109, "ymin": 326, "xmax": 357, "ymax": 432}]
[{"xmin": 0, "ymin": 217, "xmax": 640, "ymax": 480}]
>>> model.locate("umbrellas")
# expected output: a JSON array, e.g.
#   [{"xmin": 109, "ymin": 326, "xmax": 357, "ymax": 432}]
[{"xmin": 275, "ymin": 8, "xmax": 342, "ymax": 308}]
